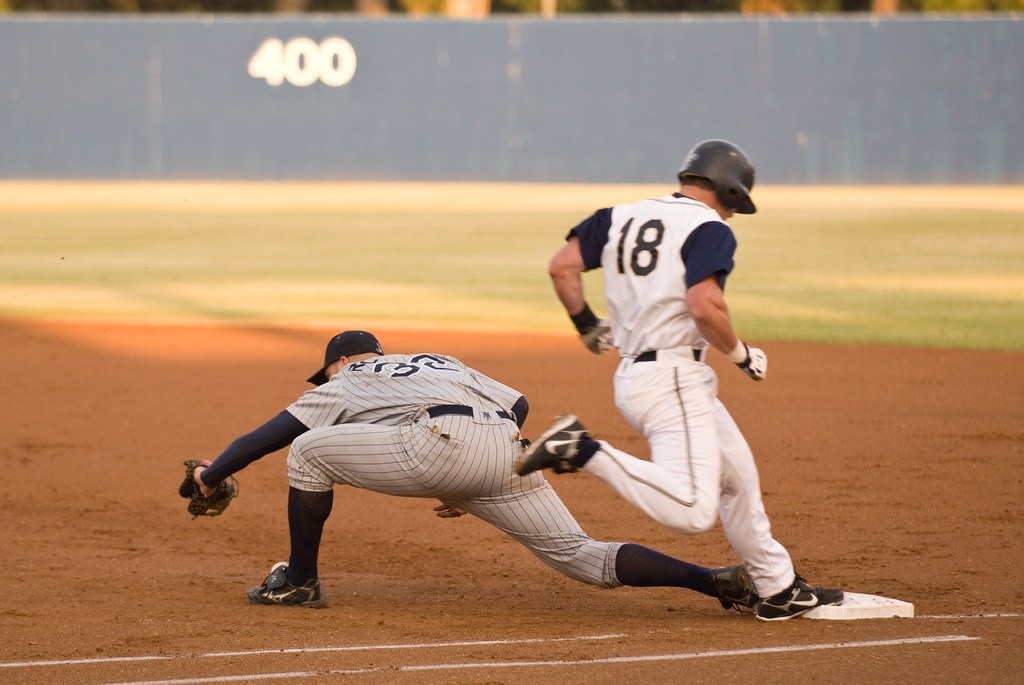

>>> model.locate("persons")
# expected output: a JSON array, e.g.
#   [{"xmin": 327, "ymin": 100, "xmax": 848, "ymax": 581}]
[
  {"xmin": 514, "ymin": 137, "xmax": 842, "ymax": 620},
  {"xmin": 180, "ymin": 330, "xmax": 807, "ymax": 609}
]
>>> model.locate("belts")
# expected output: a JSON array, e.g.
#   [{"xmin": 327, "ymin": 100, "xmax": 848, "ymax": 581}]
[
  {"xmin": 424, "ymin": 405, "xmax": 512, "ymax": 418},
  {"xmin": 633, "ymin": 349, "xmax": 702, "ymax": 362}
]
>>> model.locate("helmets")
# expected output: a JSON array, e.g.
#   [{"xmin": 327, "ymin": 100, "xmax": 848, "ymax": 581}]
[{"xmin": 678, "ymin": 139, "xmax": 756, "ymax": 215}]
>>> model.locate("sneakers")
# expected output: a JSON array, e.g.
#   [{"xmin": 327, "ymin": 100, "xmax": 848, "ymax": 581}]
[
  {"xmin": 514, "ymin": 414, "xmax": 588, "ymax": 476},
  {"xmin": 756, "ymin": 573, "xmax": 844, "ymax": 622},
  {"xmin": 709, "ymin": 563, "xmax": 758, "ymax": 614},
  {"xmin": 247, "ymin": 562, "xmax": 328, "ymax": 608}
]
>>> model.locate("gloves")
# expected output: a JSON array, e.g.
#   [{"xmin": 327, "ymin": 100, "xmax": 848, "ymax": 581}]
[
  {"xmin": 579, "ymin": 318, "xmax": 619, "ymax": 356},
  {"xmin": 734, "ymin": 341, "xmax": 767, "ymax": 382}
]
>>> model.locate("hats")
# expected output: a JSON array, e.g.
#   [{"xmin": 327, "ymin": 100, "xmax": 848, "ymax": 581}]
[{"xmin": 306, "ymin": 331, "xmax": 383, "ymax": 387}]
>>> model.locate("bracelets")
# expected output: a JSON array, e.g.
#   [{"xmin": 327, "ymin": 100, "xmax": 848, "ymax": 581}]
[
  {"xmin": 570, "ymin": 302, "xmax": 600, "ymax": 335},
  {"xmin": 725, "ymin": 341, "xmax": 749, "ymax": 364}
]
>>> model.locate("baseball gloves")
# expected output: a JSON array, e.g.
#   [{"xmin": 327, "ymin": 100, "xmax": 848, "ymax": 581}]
[{"xmin": 178, "ymin": 459, "xmax": 239, "ymax": 517}]
